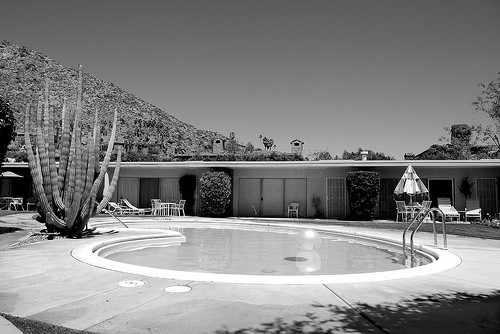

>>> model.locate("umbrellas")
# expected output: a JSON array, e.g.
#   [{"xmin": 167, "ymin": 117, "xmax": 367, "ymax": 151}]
[
  {"xmin": 393, "ymin": 164, "xmax": 429, "ymax": 206},
  {"xmin": 0, "ymin": 171, "xmax": 24, "ymax": 197}
]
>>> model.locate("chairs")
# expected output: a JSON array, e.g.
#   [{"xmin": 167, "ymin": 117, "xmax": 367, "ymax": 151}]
[
  {"xmin": 108, "ymin": 201, "xmax": 127, "ymax": 215},
  {"xmin": 287, "ymin": 202, "xmax": 300, "ymax": 218},
  {"xmin": 407, "ymin": 202, "xmax": 418, "ymax": 218},
  {"xmin": 121, "ymin": 199, "xmax": 145, "ymax": 215},
  {"xmin": 12, "ymin": 197, "xmax": 25, "ymax": 212},
  {"xmin": 464, "ymin": 197, "xmax": 483, "ymax": 222},
  {"xmin": 171, "ymin": 200, "xmax": 186, "ymax": 216},
  {"xmin": 418, "ymin": 200, "xmax": 431, "ymax": 220},
  {"xmin": 151, "ymin": 199, "xmax": 162, "ymax": 215},
  {"xmin": 437, "ymin": 197, "xmax": 460, "ymax": 221},
  {"xmin": 395, "ymin": 201, "xmax": 412, "ymax": 221},
  {"xmin": 154, "ymin": 200, "xmax": 167, "ymax": 216},
  {"xmin": 27, "ymin": 198, "xmax": 38, "ymax": 212}
]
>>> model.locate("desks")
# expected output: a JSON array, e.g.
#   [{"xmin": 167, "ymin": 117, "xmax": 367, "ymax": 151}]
[
  {"xmin": 405, "ymin": 206, "xmax": 420, "ymax": 221},
  {"xmin": 159, "ymin": 202, "xmax": 177, "ymax": 216},
  {"xmin": 1, "ymin": 197, "xmax": 15, "ymax": 211},
  {"xmin": 458, "ymin": 210, "xmax": 466, "ymax": 223}
]
